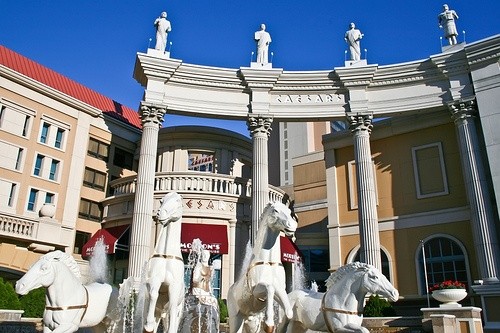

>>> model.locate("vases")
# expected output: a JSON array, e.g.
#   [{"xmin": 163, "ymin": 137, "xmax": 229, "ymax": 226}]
[{"xmin": 431, "ymin": 289, "xmax": 467, "ymax": 308}]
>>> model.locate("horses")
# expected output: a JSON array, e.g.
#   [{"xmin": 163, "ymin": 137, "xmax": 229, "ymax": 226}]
[
  {"xmin": 226, "ymin": 201, "xmax": 299, "ymax": 333},
  {"xmin": 276, "ymin": 262, "xmax": 399, "ymax": 333},
  {"xmin": 15, "ymin": 250, "xmax": 120, "ymax": 333},
  {"xmin": 143, "ymin": 191, "xmax": 185, "ymax": 333}
]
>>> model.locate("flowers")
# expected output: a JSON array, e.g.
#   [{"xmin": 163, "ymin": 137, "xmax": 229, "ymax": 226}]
[{"xmin": 428, "ymin": 280, "xmax": 467, "ymax": 294}]
